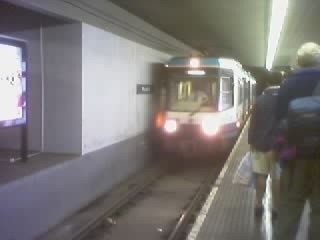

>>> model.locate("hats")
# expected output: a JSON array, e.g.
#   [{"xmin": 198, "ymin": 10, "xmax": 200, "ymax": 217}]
[{"xmin": 295, "ymin": 40, "xmax": 320, "ymax": 66}]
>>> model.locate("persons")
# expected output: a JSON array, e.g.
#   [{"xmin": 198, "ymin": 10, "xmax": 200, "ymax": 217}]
[
  {"xmin": 248, "ymin": 70, "xmax": 283, "ymax": 219},
  {"xmin": 272, "ymin": 42, "xmax": 320, "ymax": 240}
]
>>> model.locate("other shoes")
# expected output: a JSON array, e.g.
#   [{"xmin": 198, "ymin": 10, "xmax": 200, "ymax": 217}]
[{"xmin": 255, "ymin": 202, "xmax": 264, "ymax": 215}]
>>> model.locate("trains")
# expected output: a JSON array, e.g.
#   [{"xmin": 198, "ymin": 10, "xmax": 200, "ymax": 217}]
[{"xmin": 149, "ymin": 53, "xmax": 257, "ymax": 164}]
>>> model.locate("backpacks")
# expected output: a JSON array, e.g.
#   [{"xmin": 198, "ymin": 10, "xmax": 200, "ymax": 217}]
[{"xmin": 285, "ymin": 93, "xmax": 320, "ymax": 161}]
